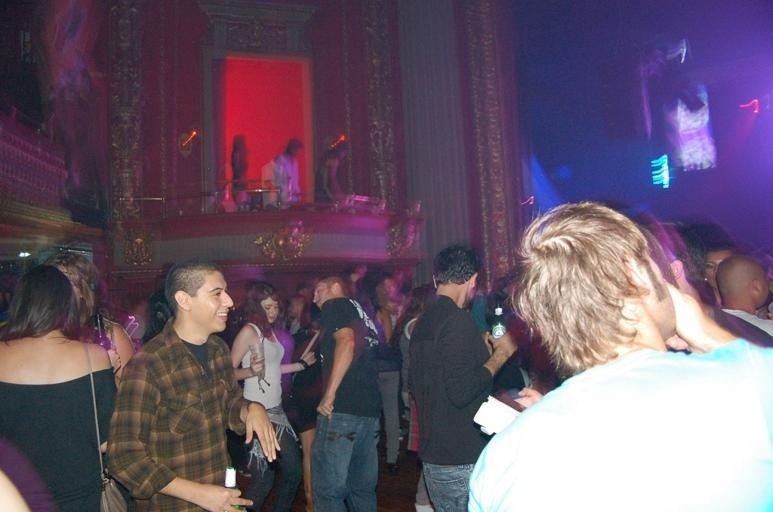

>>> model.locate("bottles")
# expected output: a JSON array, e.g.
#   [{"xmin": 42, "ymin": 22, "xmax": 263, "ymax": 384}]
[
  {"xmin": 224, "ymin": 464, "xmax": 240, "ymax": 510},
  {"xmin": 491, "ymin": 304, "xmax": 507, "ymax": 339},
  {"xmin": 95, "ymin": 313, "xmax": 114, "ymax": 369}
]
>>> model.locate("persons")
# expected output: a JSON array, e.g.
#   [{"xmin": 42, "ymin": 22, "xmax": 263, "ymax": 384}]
[
  {"xmin": 320, "ymin": 144, "xmax": 348, "ymax": 203},
  {"xmin": 272, "ymin": 138, "xmax": 305, "ymax": 209},
  {"xmin": 467, "ymin": 203, "xmax": 773, "ymax": 511},
  {"xmin": 231, "ymin": 134, "xmax": 249, "ymax": 210},
  {"xmin": 0, "ymin": 245, "xmax": 574, "ymax": 511},
  {"xmin": 260, "ymin": 155, "xmax": 279, "ymax": 208}
]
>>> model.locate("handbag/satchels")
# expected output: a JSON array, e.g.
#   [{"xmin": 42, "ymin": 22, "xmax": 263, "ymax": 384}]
[{"xmin": 100, "ymin": 479, "xmax": 128, "ymax": 512}]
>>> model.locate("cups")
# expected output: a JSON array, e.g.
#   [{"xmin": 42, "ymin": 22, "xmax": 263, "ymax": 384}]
[{"xmin": 249, "ymin": 343, "xmax": 265, "ymax": 365}]
